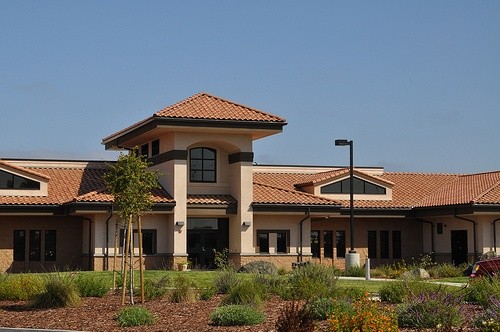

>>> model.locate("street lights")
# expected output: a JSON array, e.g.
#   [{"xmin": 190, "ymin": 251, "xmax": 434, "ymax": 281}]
[{"xmin": 335, "ymin": 139, "xmax": 357, "ymax": 254}]
